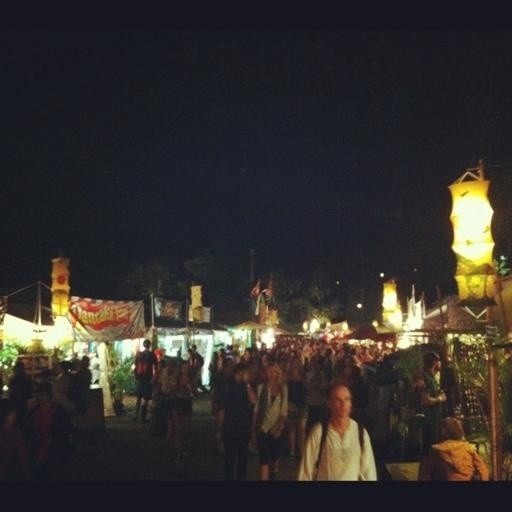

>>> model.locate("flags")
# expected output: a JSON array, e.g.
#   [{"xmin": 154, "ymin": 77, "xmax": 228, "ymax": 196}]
[
  {"xmin": 248, "ymin": 278, "xmax": 261, "ymax": 301},
  {"xmin": 261, "ymin": 274, "xmax": 277, "ymax": 297}
]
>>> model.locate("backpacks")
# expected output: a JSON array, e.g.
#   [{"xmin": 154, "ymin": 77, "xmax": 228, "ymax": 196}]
[{"xmin": 135, "ymin": 352, "xmax": 153, "ymax": 377}]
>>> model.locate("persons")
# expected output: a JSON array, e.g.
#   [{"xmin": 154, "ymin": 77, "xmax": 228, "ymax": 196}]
[{"xmin": 0, "ymin": 330, "xmax": 489, "ymax": 480}]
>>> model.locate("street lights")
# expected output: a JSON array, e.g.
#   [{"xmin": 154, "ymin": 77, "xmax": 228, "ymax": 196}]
[
  {"xmin": 448, "ymin": 160, "xmax": 502, "ymax": 481},
  {"xmin": 185, "ymin": 279, "xmax": 202, "ymax": 348},
  {"xmin": 51, "ymin": 250, "xmax": 71, "ymax": 367},
  {"xmin": 382, "ymin": 276, "xmax": 397, "ymax": 353}
]
